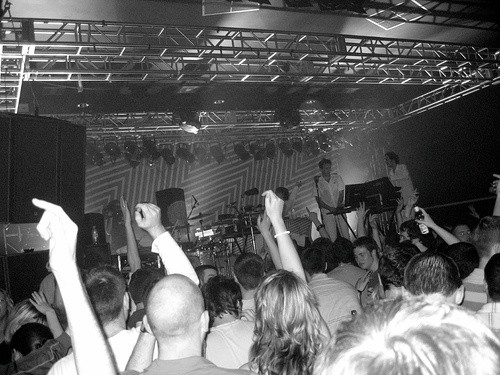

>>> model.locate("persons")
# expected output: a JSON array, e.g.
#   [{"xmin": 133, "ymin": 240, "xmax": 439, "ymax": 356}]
[
  {"xmin": 0, "ymin": 173, "xmax": 500, "ymax": 375},
  {"xmin": 385, "ymin": 152, "xmax": 417, "ymax": 227},
  {"xmin": 275, "ymin": 182, "xmax": 302, "ymax": 217},
  {"xmin": 317, "ymin": 159, "xmax": 350, "ymax": 241}
]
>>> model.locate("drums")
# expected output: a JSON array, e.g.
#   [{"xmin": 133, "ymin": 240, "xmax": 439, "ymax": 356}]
[
  {"xmin": 237, "ymin": 213, "xmax": 261, "ymax": 235},
  {"xmin": 212, "ymin": 218, "xmax": 244, "ymax": 243},
  {"xmin": 186, "ymin": 244, "xmax": 229, "ymax": 266}
]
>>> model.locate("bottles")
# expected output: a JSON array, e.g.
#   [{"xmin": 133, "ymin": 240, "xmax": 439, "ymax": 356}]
[
  {"xmin": 91, "ymin": 225, "xmax": 99, "ymax": 245},
  {"xmin": 291, "ymin": 209, "xmax": 296, "ymax": 220},
  {"xmin": 414, "ymin": 206, "xmax": 430, "ymax": 236}
]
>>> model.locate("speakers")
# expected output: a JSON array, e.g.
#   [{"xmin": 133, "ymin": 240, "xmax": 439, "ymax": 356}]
[
  {"xmin": 156, "ymin": 187, "xmax": 188, "ymax": 230},
  {"xmin": 0, "ymin": 110, "xmax": 113, "ymax": 306}
]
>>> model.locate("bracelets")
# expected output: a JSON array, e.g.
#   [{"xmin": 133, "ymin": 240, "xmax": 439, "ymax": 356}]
[
  {"xmin": 274, "ymin": 231, "xmax": 290, "ymax": 239},
  {"xmin": 316, "ymin": 224, "xmax": 325, "ymax": 231}
]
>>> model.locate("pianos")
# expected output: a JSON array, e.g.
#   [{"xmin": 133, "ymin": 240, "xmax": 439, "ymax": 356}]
[{"xmin": 345, "ymin": 176, "xmax": 397, "ymax": 235}]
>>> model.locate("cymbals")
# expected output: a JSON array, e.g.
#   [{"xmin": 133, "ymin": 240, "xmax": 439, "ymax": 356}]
[
  {"xmin": 188, "ymin": 213, "xmax": 213, "ymax": 220},
  {"xmin": 170, "ymin": 224, "xmax": 195, "ymax": 230}
]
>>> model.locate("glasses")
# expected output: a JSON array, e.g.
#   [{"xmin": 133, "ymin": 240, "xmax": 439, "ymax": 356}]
[{"xmin": 456, "ymin": 230, "xmax": 472, "ymax": 239}]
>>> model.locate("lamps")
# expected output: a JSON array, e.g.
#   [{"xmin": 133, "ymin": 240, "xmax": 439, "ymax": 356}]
[
  {"xmin": 75, "ymin": 93, "xmax": 90, "ymax": 109},
  {"xmin": 211, "ymin": 89, "xmax": 224, "ymax": 105},
  {"xmin": 177, "ymin": 109, "xmax": 201, "ymax": 135},
  {"xmin": 274, "ymin": 105, "xmax": 302, "ymax": 130},
  {"xmin": 84, "ymin": 131, "xmax": 373, "ymax": 167}
]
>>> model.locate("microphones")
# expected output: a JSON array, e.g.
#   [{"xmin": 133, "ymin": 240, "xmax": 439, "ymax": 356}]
[
  {"xmin": 226, "ymin": 202, "xmax": 237, "ymax": 207},
  {"xmin": 192, "ymin": 195, "xmax": 199, "ymax": 205}
]
